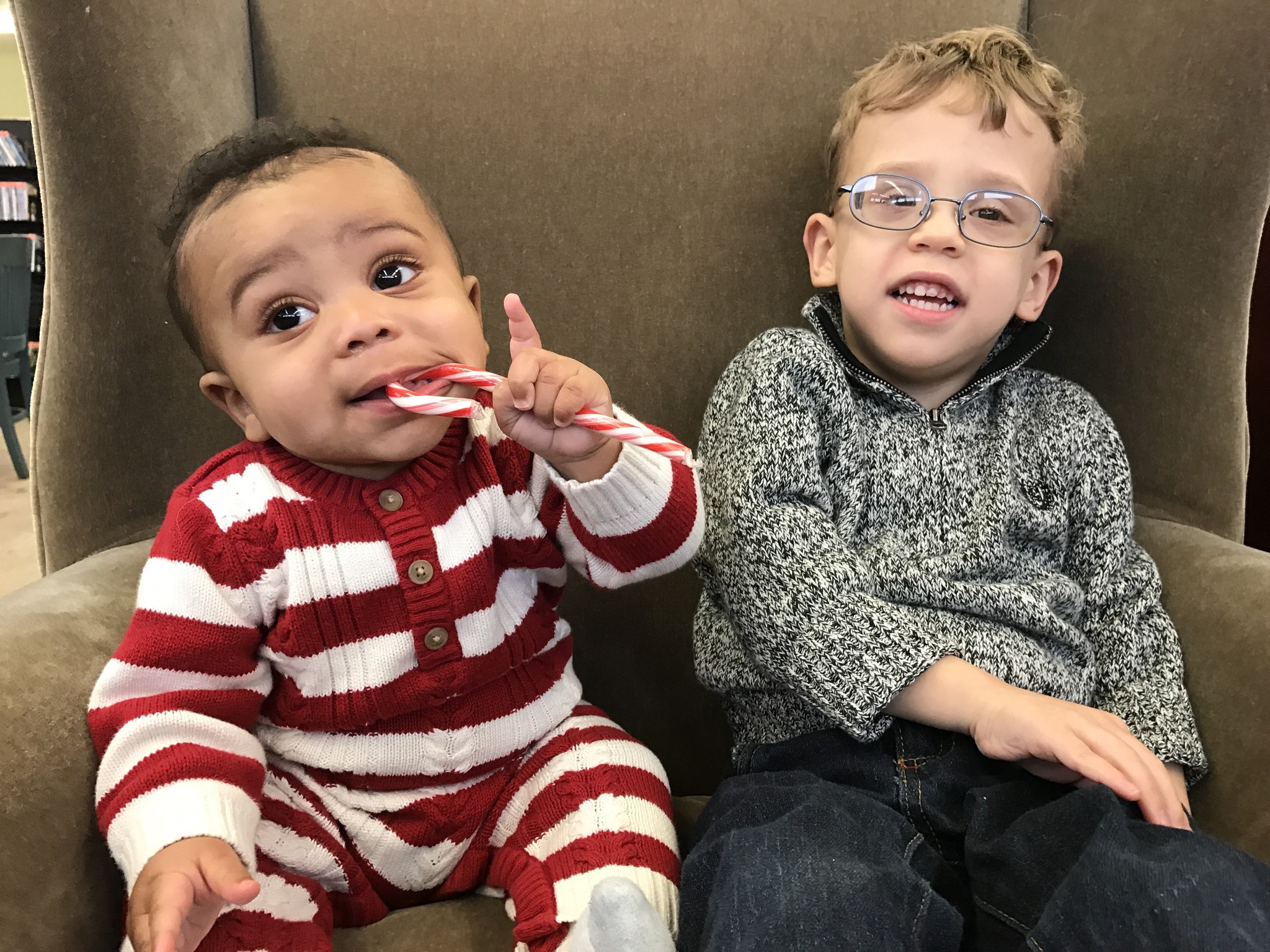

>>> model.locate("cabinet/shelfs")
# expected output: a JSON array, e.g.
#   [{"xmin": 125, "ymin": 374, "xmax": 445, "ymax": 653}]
[{"xmin": 1, "ymin": 116, "xmax": 46, "ymax": 379}]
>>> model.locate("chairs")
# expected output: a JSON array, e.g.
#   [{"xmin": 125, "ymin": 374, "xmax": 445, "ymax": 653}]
[{"xmin": 0, "ymin": 231, "xmax": 34, "ymax": 485}]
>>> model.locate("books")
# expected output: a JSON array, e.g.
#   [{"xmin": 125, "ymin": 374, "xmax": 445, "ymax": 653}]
[{"xmin": 0, "ymin": 129, "xmax": 50, "ymax": 275}]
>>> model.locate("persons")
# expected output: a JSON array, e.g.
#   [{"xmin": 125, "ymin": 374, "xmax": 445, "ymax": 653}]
[
  {"xmin": 87, "ymin": 112, "xmax": 706, "ymax": 952},
  {"xmin": 690, "ymin": 22, "xmax": 1268, "ymax": 952}
]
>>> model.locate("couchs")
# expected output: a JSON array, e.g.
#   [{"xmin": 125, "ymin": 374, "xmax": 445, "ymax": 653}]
[{"xmin": 0, "ymin": 0, "xmax": 1270, "ymax": 950}]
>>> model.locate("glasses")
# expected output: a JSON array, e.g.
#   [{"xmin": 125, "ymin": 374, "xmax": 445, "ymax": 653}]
[{"xmin": 825, "ymin": 175, "xmax": 1055, "ymax": 249}]
